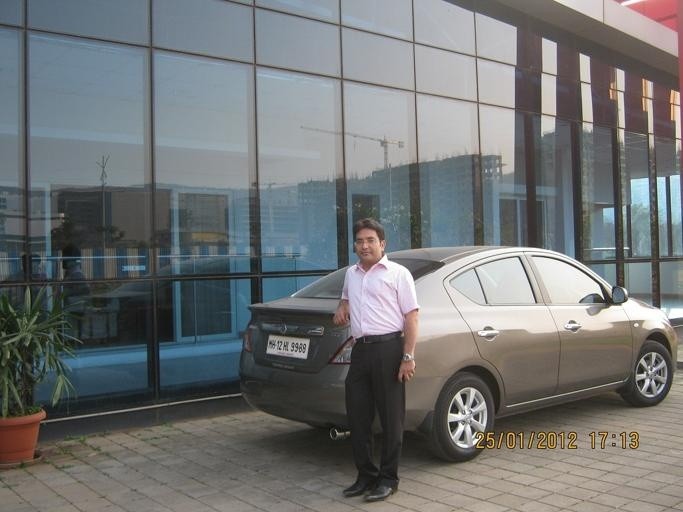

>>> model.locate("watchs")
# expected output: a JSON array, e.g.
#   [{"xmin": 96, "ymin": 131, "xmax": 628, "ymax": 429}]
[{"xmin": 400, "ymin": 352, "xmax": 414, "ymax": 363}]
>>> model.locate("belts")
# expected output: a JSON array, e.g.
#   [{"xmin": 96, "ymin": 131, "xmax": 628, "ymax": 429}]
[{"xmin": 355, "ymin": 331, "xmax": 401, "ymax": 345}]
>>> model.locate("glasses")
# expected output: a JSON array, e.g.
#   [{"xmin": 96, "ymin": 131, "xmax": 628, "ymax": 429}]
[{"xmin": 352, "ymin": 238, "xmax": 380, "ymax": 246}]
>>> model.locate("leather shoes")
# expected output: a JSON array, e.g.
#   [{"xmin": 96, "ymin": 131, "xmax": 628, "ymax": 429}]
[
  {"xmin": 364, "ymin": 484, "xmax": 397, "ymax": 503},
  {"xmin": 343, "ymin": 479, "xmax": 376, "ymax": 496}
]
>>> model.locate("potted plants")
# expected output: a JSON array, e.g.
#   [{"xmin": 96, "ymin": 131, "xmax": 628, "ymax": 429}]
[{"xmin": 0, "ymin": 277, "xmax": 89, "ymax": 464}]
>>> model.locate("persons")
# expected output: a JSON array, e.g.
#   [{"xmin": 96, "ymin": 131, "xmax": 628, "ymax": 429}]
[
  {"xmin": 331, "ymin": 216, "xmax": 421, "ymax": 502},
  {"xmin": 48, "ymin": 245, "xmax": 90, "ymax": 351},
  {"xmin": 2, "ymin": 251, "xmax": 47, "ymax": 310}
]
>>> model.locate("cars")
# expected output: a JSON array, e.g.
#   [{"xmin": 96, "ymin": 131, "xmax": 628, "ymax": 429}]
[
  {"xmin": 79, "ymin": 255, "xmax": 327, "ymax": 355},
  {"xmin": 237, "ymin": 241, "xmax": 679, "ymax": 464}
]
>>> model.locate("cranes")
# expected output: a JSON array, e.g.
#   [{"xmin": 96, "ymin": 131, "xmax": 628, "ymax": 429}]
[{"xmin": 298, "ymin": 123, "xmax": 404, "ymax": 181}]
